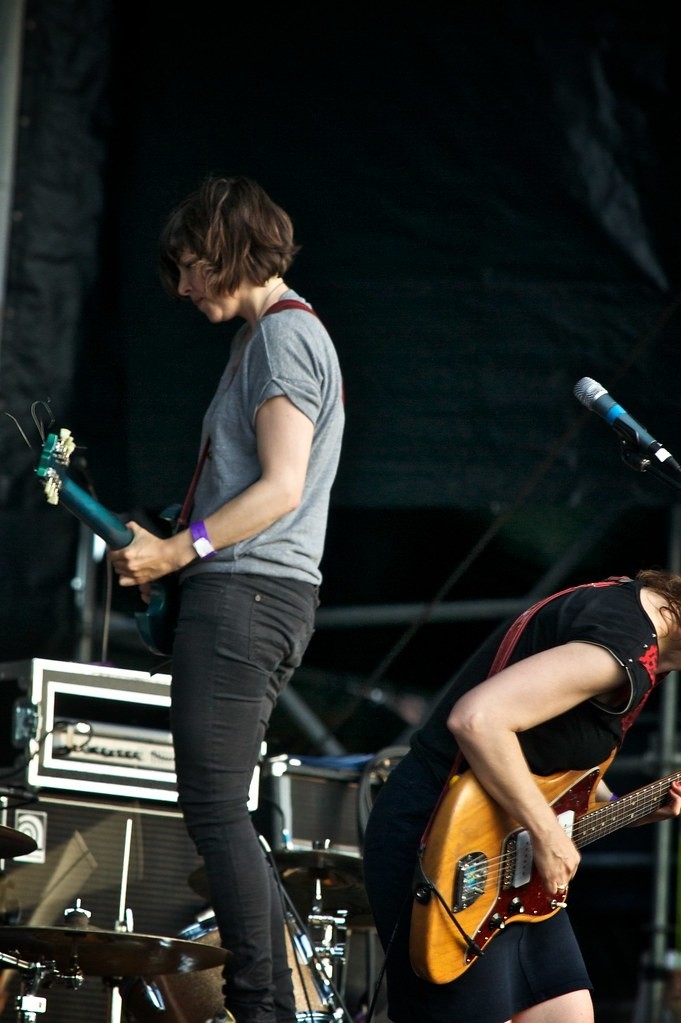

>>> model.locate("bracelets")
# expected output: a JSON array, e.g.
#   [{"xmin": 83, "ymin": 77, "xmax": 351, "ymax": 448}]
[
  {"xmin": 189, "ymin": 520, "xmax": 218, "ymax": 559},
  {"xmin": 610, "ymin": 794, "xmax": 619, "ymax": 801}
]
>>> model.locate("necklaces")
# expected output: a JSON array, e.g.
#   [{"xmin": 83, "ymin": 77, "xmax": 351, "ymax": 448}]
[{"xmin": 257, "ymin": 279, "xmax": 283, "ymax": 317}]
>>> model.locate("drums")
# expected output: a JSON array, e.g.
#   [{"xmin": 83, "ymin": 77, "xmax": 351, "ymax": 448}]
[{"xmin": 146, "ymin": 907, "xmax": 323, "ymax": 1023}]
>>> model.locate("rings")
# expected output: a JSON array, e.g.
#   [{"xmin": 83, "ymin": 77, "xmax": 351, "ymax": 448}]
[{"xmin": 558, "ymin": 885, "xmax": 565, "ymax": 890}]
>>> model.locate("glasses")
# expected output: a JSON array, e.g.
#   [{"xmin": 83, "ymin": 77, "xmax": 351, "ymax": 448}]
[{"xmin": 168, "ymin": 255, "xmax": 206, "ymax": 285}]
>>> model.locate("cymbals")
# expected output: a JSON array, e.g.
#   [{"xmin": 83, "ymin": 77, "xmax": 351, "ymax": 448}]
[
  {"xmin": 269, "ymin": 845, "xmax": 373, "ymax": 927},
  {"xmin": 0, "ymin": 824, "xmax": 38, "ymax": 859},
  {"xmin": 1, "ymin": 921, "xmax": 228, "ymax": 975}
]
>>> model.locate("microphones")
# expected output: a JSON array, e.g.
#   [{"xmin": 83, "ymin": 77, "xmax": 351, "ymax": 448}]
[{"xmin": 575, "ymin": 377, "xmax": 681, "ymax": 473}]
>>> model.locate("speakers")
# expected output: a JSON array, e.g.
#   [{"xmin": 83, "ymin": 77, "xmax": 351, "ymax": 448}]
[{"xmin": 0, "ymin": 788, "xmax": 216, "ymax": 1023}]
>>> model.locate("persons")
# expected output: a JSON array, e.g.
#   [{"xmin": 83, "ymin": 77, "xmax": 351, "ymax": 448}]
[
  {"xmin": 362, "ymin": 572, "xmax": 681, "ymax": 1023},
  {"xmin": 108, "ymin": 176, "xmax": 345, "ymax": 1023}
]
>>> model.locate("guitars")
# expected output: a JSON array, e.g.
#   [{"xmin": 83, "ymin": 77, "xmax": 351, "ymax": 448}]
[
  {"xmin": 408, "ymin": 745, "xmax": 681, "ymax": 986},
  {"xmin": 31, "ymin": 426, "xmax": 186, "ymax": 659}
]
